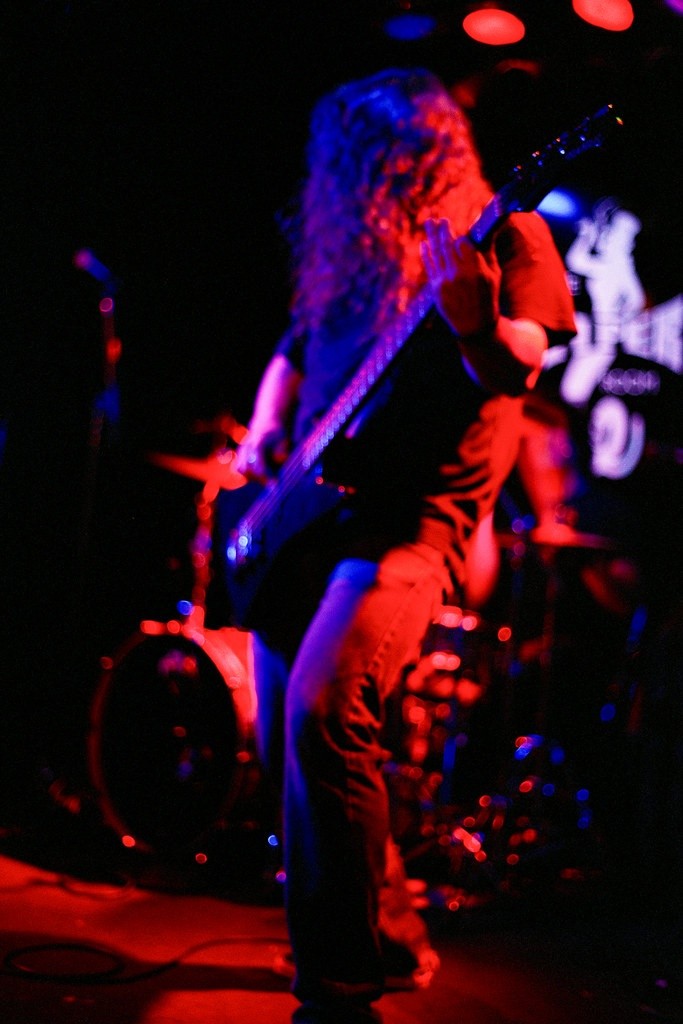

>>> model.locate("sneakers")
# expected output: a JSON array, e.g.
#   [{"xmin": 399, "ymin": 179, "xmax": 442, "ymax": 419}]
[
  {"xmin": 287, "ymin": 1003, "xmax": 385, "ymax": 1023},
  {"xmin": 272, "ymin": 936, "xmax": 442, "ymax": 989}
]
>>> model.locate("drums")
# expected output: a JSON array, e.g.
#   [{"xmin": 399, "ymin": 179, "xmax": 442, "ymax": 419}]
[{"xmin": 89, "ymin": 617, "xmax": 292, "ymax": 860}]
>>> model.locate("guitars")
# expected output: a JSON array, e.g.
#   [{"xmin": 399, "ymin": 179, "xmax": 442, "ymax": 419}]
[{"xmin": 201, "ymin": 98, "xmax": 628, "ymax": 636}]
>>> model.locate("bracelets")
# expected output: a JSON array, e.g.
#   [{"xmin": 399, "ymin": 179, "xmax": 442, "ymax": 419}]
[{"xmin": 456, "ymin": 318, "xmax": 499, "ymax": 348}]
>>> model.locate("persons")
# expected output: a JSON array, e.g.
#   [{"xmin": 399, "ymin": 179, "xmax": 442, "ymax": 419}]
[{"xmin": 235, "ymin": 70, "xmax": 577, "ymax": 1024}]
